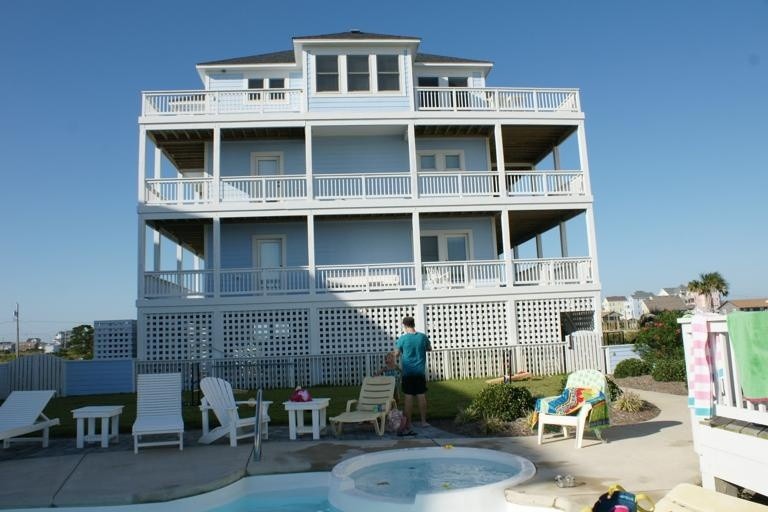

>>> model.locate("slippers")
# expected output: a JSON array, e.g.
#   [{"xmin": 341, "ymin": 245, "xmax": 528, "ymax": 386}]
[{"xmin": 401, "ymin": 430, "xmax": 418, "ymax": 436}]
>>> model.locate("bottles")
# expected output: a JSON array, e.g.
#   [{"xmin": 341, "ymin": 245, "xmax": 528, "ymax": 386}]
[
  {"xmin": 374, "ymin": 403, "xmax": 381, "ymax": 413},
  {"xmin": 555, "ymin": 474, "xmax": 576, "ymax": 488}
]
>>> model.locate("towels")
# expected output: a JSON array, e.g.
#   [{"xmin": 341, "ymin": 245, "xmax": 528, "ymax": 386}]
[{"xmin": 685, "ymin": 309, "xmax": 768, "ymax": 420}]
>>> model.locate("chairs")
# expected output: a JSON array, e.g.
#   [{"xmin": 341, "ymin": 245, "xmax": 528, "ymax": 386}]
[
  {"xmin": 1, "ymin": 390, "xmax": 61, "ymax": 448},
  {"xmin": 131, "ymin": 372, "xmax": 185, "ymax": 454},
  {"xmin": 196, "ymin": 375, "xmax": 274, "ymax": 448},
  {"xmin": 328, "ymin": 375, "xmax": 398, "ymax": 436},
  {"xmin": 536, "ymin": 368, "xmax": 611, "ymax": 449}
]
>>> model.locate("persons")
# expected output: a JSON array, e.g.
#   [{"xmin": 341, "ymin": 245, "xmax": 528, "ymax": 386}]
[
  {"xmin": 378, "ymin": 351, "xmax": 402, "ymax": 403},
  {"xmin": 392, "ymin": 316, "xmax": 433, "ymax": 428}
]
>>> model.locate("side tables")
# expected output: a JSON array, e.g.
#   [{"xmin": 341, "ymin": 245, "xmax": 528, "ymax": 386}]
[
  {"xmin": 70, "ymin": 404, "xmax": 126, "ymax": 449},
  {"xmin": 282, "ymin": 397, "xmax": 331, "ymax": 440}
]
